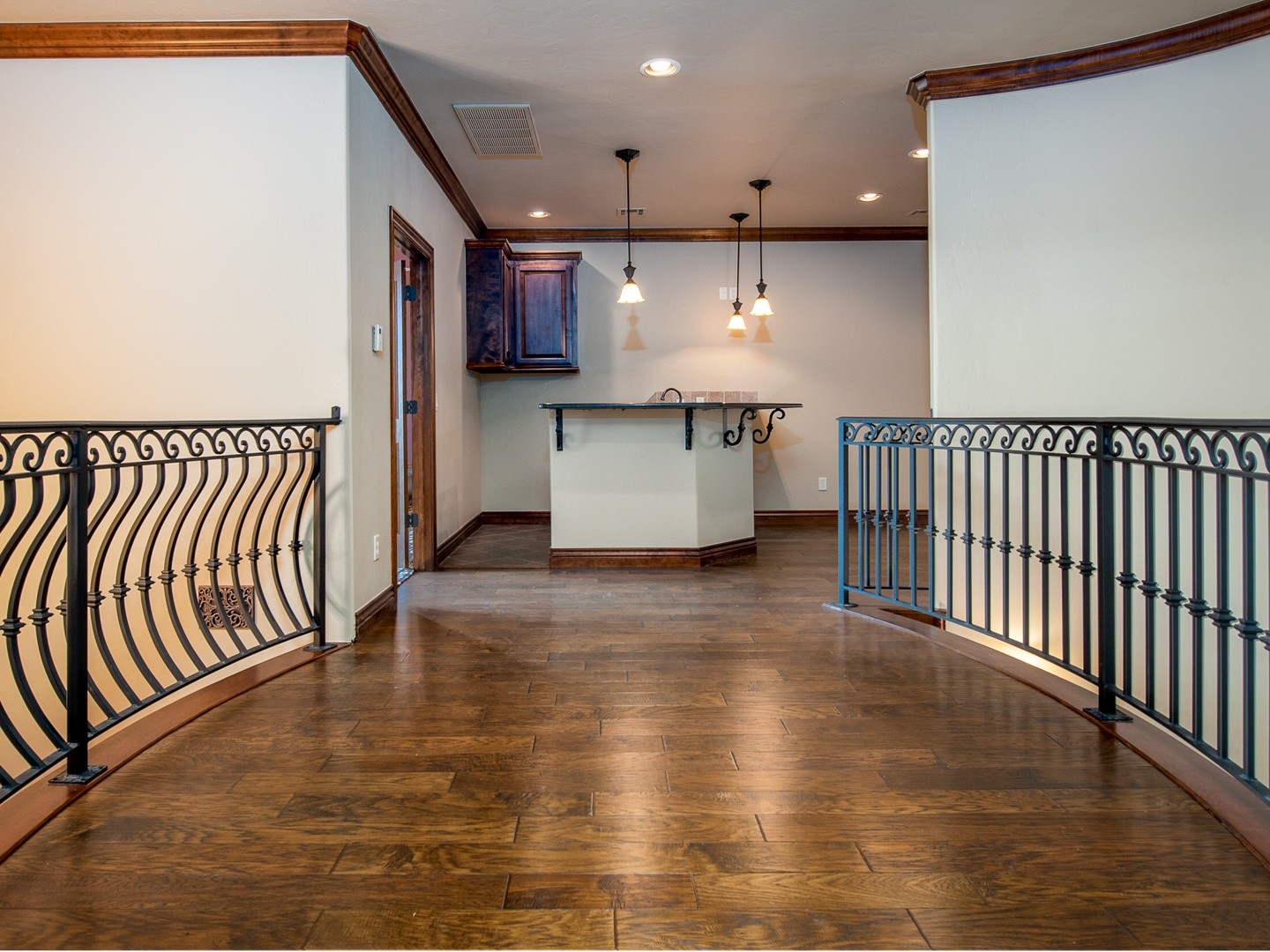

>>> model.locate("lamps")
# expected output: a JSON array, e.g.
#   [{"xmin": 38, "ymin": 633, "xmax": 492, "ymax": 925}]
[
  {"xmin": 613, "ymin": 148, "xmax": 646, "ymax": 305},
  {"xmin": 747, "ymin": 179, "xmax": 775, "ymax": 317},
  {"xmin": 726, "ymin": 212, "xmax": 749, "ymax": 330}
]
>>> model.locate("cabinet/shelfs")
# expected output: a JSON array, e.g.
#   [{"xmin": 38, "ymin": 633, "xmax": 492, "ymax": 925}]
[{"xmin": 464, "ymin": 236, "xmax": 585, "ymax": 377}]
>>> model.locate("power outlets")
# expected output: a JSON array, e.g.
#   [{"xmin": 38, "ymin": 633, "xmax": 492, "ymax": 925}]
[{"xmin": 818, "ymin": 477, "xmax": 829, "ymax": 491}]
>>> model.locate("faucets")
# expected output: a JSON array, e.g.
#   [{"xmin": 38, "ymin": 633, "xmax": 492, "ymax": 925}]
[{"xmin": 660, "ymin": 388, "xmax": 683, "ymax": 403}]
